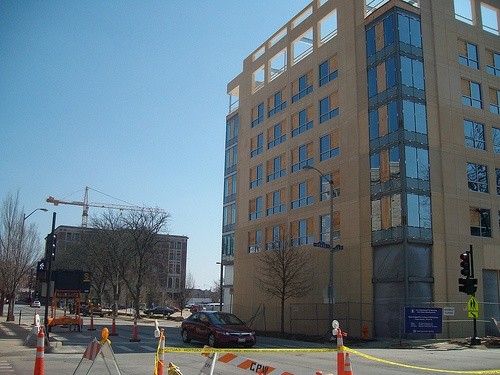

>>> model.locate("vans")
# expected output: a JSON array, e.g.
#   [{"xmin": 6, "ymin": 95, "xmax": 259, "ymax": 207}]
[{"xmin": 139, "ymin": 303, "xmax": 146, "ymax": 310}]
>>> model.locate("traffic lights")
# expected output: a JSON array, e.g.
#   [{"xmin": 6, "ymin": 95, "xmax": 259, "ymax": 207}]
[
  {"xmin": 460, "ymin": 251, "xmax": 470, "ymax": 276},
  {"xmin": 467, "ymin": 279, "xmax": 477, "ymax": 295},
  {"xmin": 37, "ymin": 261, "xmax": 47, "ymax": 281},
  {"xmin": 458, "ymin": 277, "xmax": 467, "ymax": 294}
]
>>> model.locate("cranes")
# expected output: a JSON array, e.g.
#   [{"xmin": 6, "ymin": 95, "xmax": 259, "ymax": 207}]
[{"xmin": 46, "ymin": 186, "xmax": 166, "ymax": 228}]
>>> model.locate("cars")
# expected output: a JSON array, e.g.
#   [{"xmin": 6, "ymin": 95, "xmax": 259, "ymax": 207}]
[
  {"xmin": 169, "ymin": 306, "xmax": 180, "ymax": 312},
  {"xmin": 30, "ymin": 300, "xmax": 40, "ymax": 308},
  {"xmin": 180, "ymin": 311, "xmax": 257, "ymax": 349},
  {"xmin": 57, "ymin": 297, "xmax": 104, "ymax": 317},
  {"xmin": 143, "ymin": 305, "xmax": 175, "ymax": 316}
]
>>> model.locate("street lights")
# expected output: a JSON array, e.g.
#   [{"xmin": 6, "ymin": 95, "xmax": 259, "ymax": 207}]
[
  {"xmin": 11, "ymin": 207, "xmax": 48, "ymax": 314},
  {"xmin": 301, "ymin": 165, "xmax": 334, "ymax": 340},
  {"xmin": 216, "ymin": 262, "xmax": 223, "ymax": 311}
]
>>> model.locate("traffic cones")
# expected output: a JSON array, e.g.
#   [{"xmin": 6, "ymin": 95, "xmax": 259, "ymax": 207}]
[
  {"xmin": 362, "ymin": 325, "xmax": 369, "ymax": 339},
  {"xmin": 343, "ymin": 352, "xmax": 353, "ymax": 375}
]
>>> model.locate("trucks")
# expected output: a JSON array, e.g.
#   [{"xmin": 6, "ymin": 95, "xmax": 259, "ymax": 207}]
[{"xmin": 190, "ymin": 304, "xmax": 215, "ymax": 313}]
